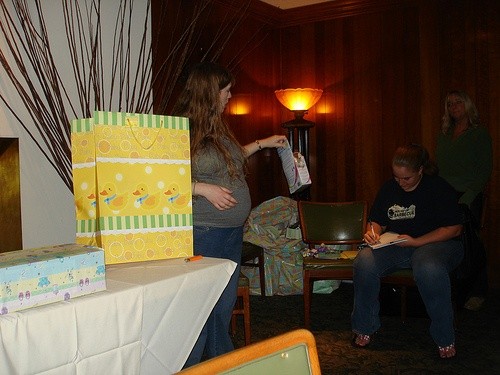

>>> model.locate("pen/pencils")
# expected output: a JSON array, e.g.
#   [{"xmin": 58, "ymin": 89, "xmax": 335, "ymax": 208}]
[{"xmin": 370, "ymin": 223, "xmax": 376, "ymax": 239}]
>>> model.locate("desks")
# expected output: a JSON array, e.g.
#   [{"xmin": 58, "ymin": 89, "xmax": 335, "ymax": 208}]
[{"xmin": 0, "ymin": 241, "xmax": 243, "ymax": 375}]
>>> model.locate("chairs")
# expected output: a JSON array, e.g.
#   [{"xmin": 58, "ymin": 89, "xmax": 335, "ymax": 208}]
[
  {"xmin": 296, "ymin": 198, "xmax": 370, "ymax": 330},
  {"xmin": 381, "ymin": 270, "xmax": 460, "ymax": 334},
  {"xmin": 229, "ymin": 238, "xmax": 270, "ymax": 347}
]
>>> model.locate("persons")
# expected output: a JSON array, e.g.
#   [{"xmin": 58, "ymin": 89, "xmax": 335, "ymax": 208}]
[
  {"xmin": 169, "ymin": 62, "xmax": 289, "ymax": 370},
  {"xmin": 352, "ymin": 146, "xmax": 465, "ymax": 361},
  {"xmin": 436, "ymin": 89, "xmax": 492, "ymax": 312}
]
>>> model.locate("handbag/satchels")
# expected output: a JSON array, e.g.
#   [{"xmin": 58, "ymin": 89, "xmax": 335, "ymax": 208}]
[{"xmin": 69, "ymin": 110, "xmax": 194, "ymax": 264}]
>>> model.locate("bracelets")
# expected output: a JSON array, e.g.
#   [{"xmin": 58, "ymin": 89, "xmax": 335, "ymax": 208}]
[{"xmin": 256, "ymin": 140, "xmax": 262, "ymax": 150}]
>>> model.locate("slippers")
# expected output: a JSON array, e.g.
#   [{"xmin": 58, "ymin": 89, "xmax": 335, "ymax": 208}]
[
  {"xmin": 437, "ymin": 341, "xmax": 457, "ymax": 365},
  {"xmin": 352, "ymin": 332, "xmax": 378, "ymax": 348}
]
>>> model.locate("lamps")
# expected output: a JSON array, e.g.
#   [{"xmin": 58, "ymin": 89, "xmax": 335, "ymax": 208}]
[{"xmin": 274, "ymin": 86, "xmax": 325, "ymax": 198}]
